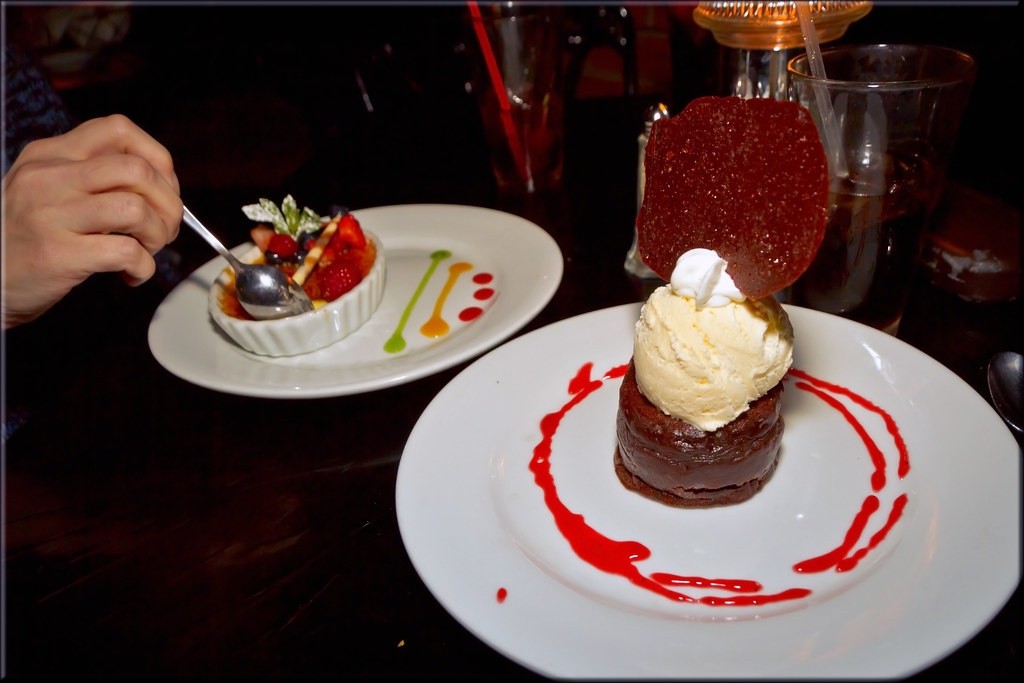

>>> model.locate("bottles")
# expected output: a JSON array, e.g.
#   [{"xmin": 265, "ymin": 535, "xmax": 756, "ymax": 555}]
[{"xmin": 691, "ymin": 0, "xmax": 874, "ymax": 103}]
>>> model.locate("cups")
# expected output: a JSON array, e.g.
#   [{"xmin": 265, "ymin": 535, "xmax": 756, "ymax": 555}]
[
  {"xmin": 788, "ymin": 44, "xmax": 975, "ymax": 335},
  {"xmin": 463, "ymin": 0, "xmax": 565, "ymax": 202},
  {"xmin": 565, "ymin": 5, "xmax": 672, "ymax": 98}
]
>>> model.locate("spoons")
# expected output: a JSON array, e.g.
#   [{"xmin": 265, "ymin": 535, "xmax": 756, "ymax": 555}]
[
  {"xmin": 182, "ymin": 206, "xmax": 315, "ymax": 321},
  {"xmin": 987, "ymin": 352, "xmax": 1024, "ymax": 432}
]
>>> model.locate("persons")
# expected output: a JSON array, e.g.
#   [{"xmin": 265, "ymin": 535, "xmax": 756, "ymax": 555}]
[{"xmin": 5, "ymin": 5, "xmax": 183, "ymax": 440}]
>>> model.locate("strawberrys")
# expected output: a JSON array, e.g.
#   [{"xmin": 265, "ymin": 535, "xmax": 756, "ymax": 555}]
[
  {"xmin": 269, "ymin": 235, "xmax": 296, "ymax": 252},
  {"xmin": 321, "ymin": 258, "xmax": 359, "ymax": 300}
]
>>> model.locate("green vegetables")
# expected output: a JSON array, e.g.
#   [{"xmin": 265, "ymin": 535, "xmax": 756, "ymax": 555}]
[{"xmin": 242, "ymin": 195, "xmax": 322, "ymax": 237}]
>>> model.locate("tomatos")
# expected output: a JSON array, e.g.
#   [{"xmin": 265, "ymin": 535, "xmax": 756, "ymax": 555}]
[{"xmin": 332, "ymin": 215, "xmax": 367, "ymax": 254}]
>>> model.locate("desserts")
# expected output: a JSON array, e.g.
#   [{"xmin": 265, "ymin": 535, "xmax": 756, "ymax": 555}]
[{"xmin": 612, "ymin": 97, "xmax": 829, "ymax": 512}]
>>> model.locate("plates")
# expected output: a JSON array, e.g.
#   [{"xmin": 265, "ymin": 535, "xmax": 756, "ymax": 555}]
[
  {"xmin": 149, "ymin": 204, "xmax": 562, "ymax": 398},
  {"xmin": 207, "ymin": 231, "xmax": 387, "ymax": 357},
  {"xmin": 395, "ymin": 300, "xmax": 1019, "ymax": 678}
]
